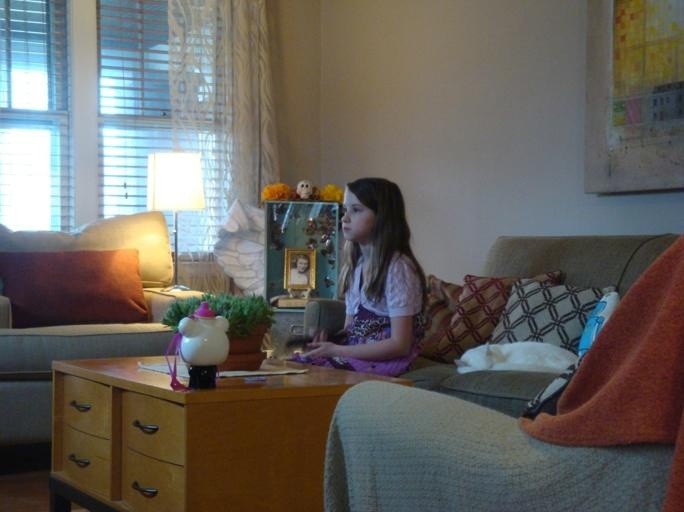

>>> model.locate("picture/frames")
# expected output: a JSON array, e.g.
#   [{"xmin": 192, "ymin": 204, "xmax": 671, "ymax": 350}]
[
  {"xmin": 284, "ymin": 247, "xmax": 317, "ymax": 290},
  {"xmin": 584, "ymin": 0, "xmax": 684, "ymax": 194}
]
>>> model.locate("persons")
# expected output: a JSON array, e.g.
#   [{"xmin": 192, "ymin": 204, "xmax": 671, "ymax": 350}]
[
  {"xmin": 283, "ymin": 177, "xmax": 427, "ymax": 376},
  {"xmin": 287, "ymin": 255, "xmax": 309, "ymax": 284}
]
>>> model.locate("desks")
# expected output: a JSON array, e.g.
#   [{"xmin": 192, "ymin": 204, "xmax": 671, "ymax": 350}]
[{"xmin": 49, "ymin": 356, "xmax": 415, "ymax": 512}]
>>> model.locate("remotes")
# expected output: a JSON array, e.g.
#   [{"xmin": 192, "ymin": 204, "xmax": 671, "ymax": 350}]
[{"xmin": 285, "ymin": 333, "xmax": 343, "ymax": 348}]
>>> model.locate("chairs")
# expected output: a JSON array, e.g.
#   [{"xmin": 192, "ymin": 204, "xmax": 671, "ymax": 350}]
[{"xmin": 323, "ymin": 235, "xmax": 684, "ymax": 512}]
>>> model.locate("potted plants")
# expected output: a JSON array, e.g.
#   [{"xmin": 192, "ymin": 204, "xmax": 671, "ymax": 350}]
[{"xmin": 160, "ymin": 292, "xmax": 276, "ymax": 371}]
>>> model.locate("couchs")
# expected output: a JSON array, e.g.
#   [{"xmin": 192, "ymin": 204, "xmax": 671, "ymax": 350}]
[
  {"xmin": 303, "ymin": 233, "xmax": 683, "ymax": 419},
  {"xmin": 0, "ymin": 211, "xmax": 216, "ymax": 447}
]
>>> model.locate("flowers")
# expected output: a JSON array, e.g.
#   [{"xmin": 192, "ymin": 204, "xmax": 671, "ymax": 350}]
[
  {"xmin": 261, "ymin": 184, "xmax": 296, "ymax": 200},
  {"xmin": 318, "ymin": 182, "xmax": 344, "ymax": 202}
]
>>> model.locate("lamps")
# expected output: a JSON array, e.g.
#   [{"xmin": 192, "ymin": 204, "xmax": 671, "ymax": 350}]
[{"xmin": 146, "ymin": 151, "xmax": 206, "ymax": 292}]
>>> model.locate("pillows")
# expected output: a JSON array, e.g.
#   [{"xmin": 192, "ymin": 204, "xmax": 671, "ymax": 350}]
[
  {"xmin": 425, "ymin": 270, "xmax": 622, "ymax": 419},
  {"xmin": 0, "ymin": 210, "xmax": 174, "ymax": 288},
  {"xmin": 0, "ymin": 249, "xmax": 153, "ymax": 328}
]
